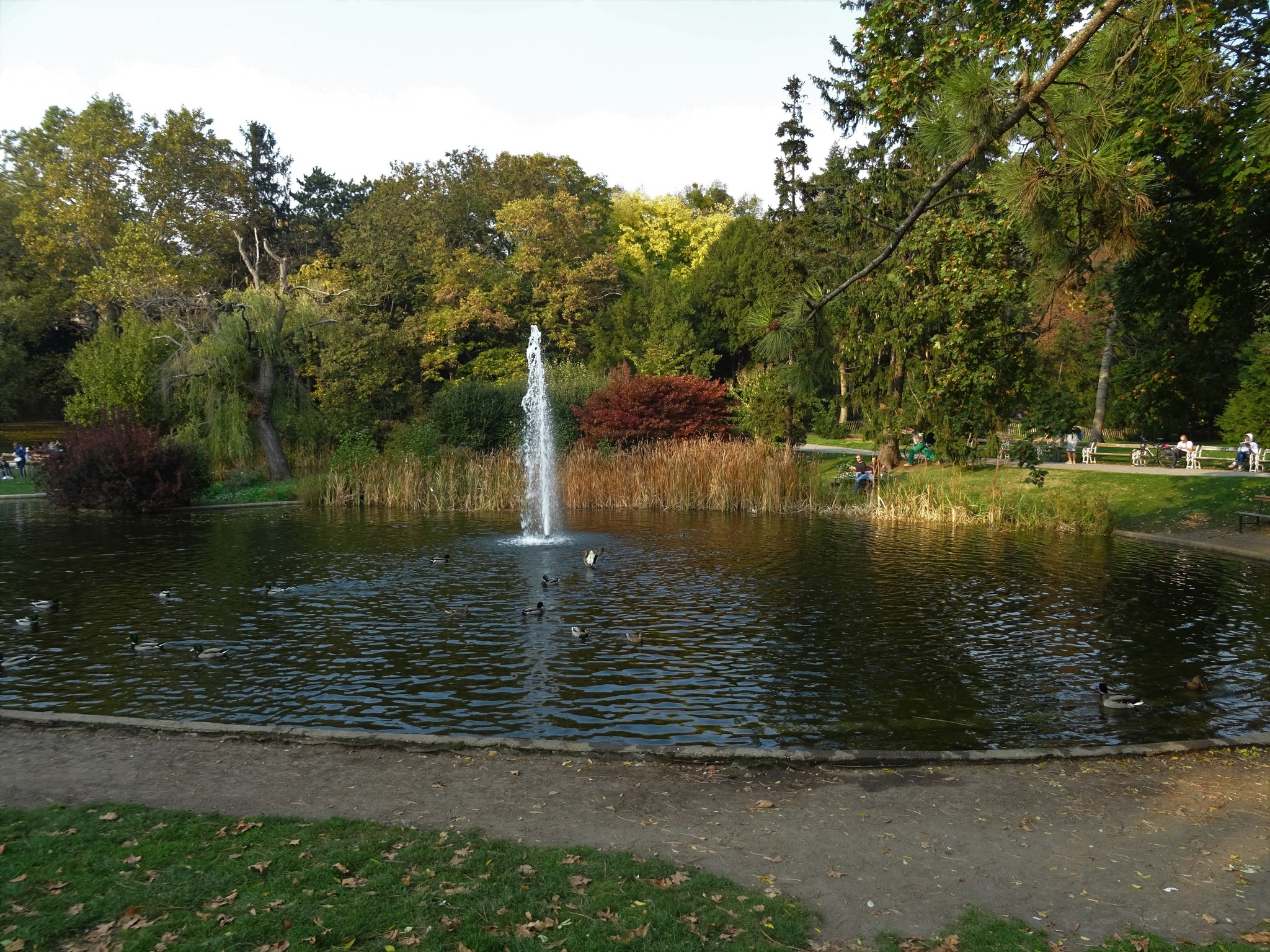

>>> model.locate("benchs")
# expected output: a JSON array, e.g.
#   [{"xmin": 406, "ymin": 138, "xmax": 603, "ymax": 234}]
[
  {"xmin": 1234, "ymin": 495, "xmax": 1270, "ymax": 533},
  {"xmin": 970, "ymin": 438, "xmax": 1270, "ymax": 473},
  {"xmin": 0, "ymin": 453, "xmax": 50, "ymax": 476},
  {"xmin": 831, "ymin": 467, "xmax": 886, "ymax": 489}
]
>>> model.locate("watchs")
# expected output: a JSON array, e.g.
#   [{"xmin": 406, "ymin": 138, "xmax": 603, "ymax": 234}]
[{"xmin": 1171, "ymin": 435, "xmax": 1193, "ymax": 468}]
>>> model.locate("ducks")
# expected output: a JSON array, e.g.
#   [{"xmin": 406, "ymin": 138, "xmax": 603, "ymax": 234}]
[
  {"xmin": 571, "ymin": 626, "xmax": 589, "ymax": 638},
  {"xmin": 540, "ymin": 575, "xmax": 560, "ymax": 585},
  {"xmin": 261, "ymin": 582, "xmax": 292, "ymax": 595},
  {"xmin": 125, "ymin": 632, "xmax": 168, "ymax": 652},
  {"xmin": 188, "ymin": 644, "xmax": 230, "ymax": 660},
  {"xmin": 15, "ymin": 613, "xmax": 44, "ymax": 627},
  {"xmin": 444, "ymin": 604, "xmax": 470, "ymax": 615},
  {"xmin": 1095, "ymin": 682, "xmax": 1146, "ymax": 709},
  {"xmin": 581, "ymin": 547, "xmax": 605, "ymax": 567},
  {"xmin": 625, "ymin": 630, "xmax": 645, "ymax": 643},
  {"xmin": 158, "ymin": 585, "xmax": 174, "ymax": 598},
  {"xmin": 521, "ymin": 601, "xmax": 546, "ymax": 615},
  {"xmin": 429, "ymin": 553, "xmax": 451, "ymax": 563},
  {"xmin": 1183, "ymin": 673, "xmax": 1212, "ymax": 693},
  {"xmin": 30, "ymin": 597, "xmax": 63, "ymax": 608}
]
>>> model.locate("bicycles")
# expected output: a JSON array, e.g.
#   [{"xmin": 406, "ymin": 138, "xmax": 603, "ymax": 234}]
[
  {"xmin": 903, "ymin": 432, "xmax": 935, "ymax": 459},
  {"xmin": 1128, "ymin": 433, "xmax": 1177, "ymax": 468}
]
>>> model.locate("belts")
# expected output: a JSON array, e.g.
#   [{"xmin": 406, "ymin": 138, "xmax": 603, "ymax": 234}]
[{"xmin": 919, "ymin": 441, "xmax": 923, "ymax": 443}]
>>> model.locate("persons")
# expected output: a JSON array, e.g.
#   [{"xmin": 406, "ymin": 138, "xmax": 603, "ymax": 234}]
[
  {"xmin": 1053, "ymin": 434, "xmax": 1065, "ymax": 462},
  {"xmin": 12, "ymin": 442, "xmax": 31, "ymax": 478},
  {"xmin": 854, "ymin": 457, "xmax": 879, "ymax": 494},
  {"xmin": 845, "ymin": 455, "xmax": 866, "ymax": 481},
  {"xmin": 38, "ymin": 439, "xmax": 67, "ymax": 451},
  {"xmin": 1228, "ymin": 434, "xmax": 1259, "ymax": 472},
  {"xmin": 0, "ymin": 460, "xmax": 12, "ymax": 479},
  {"xmin": 1066, "ymin": 434, "xmax": 1078, "ymax": 464},
  {"xmin": 901, "ymin": 425, "xmax": 940, "ymax": 467}
]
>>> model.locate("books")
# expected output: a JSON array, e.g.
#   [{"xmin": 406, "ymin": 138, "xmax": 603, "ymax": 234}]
[{"xmin": 1240, "ymin": 441, "xmax": 1252, "ymax": 448}]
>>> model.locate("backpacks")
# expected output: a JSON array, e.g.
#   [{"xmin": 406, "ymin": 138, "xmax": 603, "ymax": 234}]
[
  {"xmin": 1164, "ymin": 446, "xmax": 1176, "ymax": 456},
  {"xmin": 925, "ymin": 432, "xmax": 937, "ymax": 445},
  {"xmin": 1244, "ymin": 449, "xmax": 1255, "ymax": 465}
]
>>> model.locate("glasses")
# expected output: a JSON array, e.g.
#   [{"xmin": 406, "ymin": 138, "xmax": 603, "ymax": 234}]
[
  {"xmin": 856, "ymin": 457, "xmax": 861, "ymax": 459},
  {"xmin": 1180, "ymin": 439, "xmax": 1185, "ymax": 441}
]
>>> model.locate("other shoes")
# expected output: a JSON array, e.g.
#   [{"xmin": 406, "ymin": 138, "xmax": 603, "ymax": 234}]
[
  {"xmin": 1072, "ymin": 461, "xmax": 1075, "ymax": 464},
  {"xmin": 903, "ymin": 463, "xmax": 912, "ymax": 466},
  {"xmin": 1228, "ymin": 463, "xmax": 1235, "ymax": 468},
  {"xmin": 1065, "ymin": 461, "xmax": 1072, "ymax": 464},
  {"xmin": 1176, "ymin": 461, "xmax": 1179, "ymax": 465},
  {"xmin": 1053, "ymin": 460, "xmax": 1064, "ymax": 462},
  {"xmin": 931, "ymin": 461, "xmax": 940, "ymax": 465},
  {"xmin": 1170, "ymin": 465, "xmax": 1177, "ymax": 468},
  {"xmin": 1238, "ymin": 468, "xmax": 1242, "ymax": 472}
]
